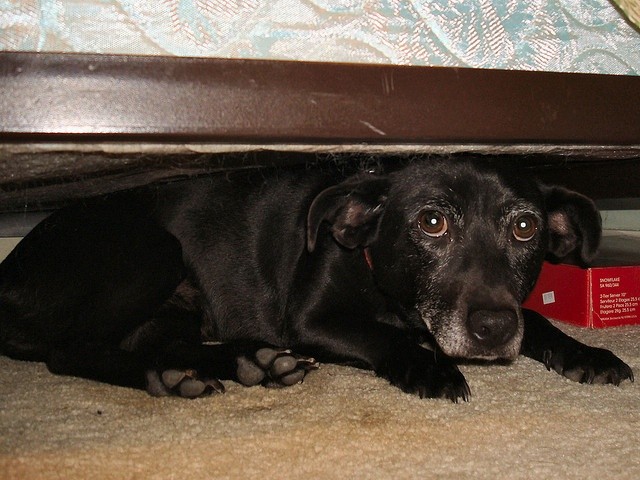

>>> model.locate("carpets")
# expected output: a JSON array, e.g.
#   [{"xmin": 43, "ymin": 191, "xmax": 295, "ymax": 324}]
[{"xmin": 1, "ymin": 227, "xmax": 638, "ymax": 479}]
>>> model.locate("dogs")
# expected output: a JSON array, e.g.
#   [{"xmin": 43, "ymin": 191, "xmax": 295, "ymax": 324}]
[{"xmin": 0, "ymin": 150, "xmax": 634, "ymax": 405}]
